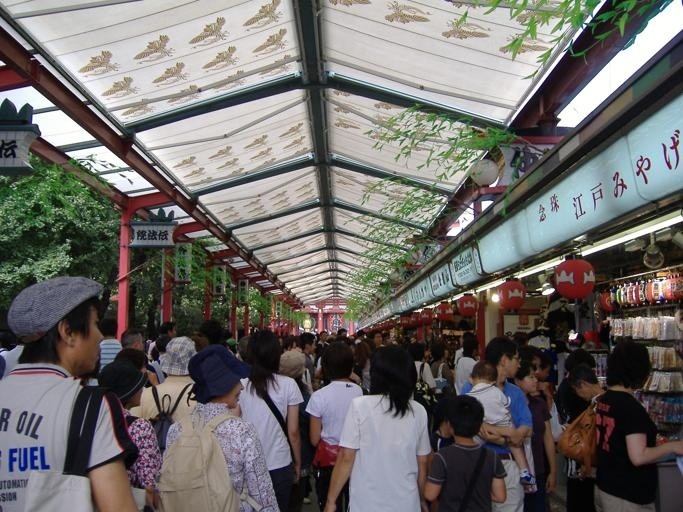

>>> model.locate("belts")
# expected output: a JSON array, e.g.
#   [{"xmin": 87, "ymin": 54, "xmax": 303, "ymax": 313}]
[{"xmin": 498, "ymin": 453, "xmax": 515, "ymax": 459}]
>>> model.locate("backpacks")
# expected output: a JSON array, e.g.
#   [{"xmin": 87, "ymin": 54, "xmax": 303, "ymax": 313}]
[
  {"xmin": 154, "ymin": 413, "xmax": 239, "ymax": 511},
  {"xmin": 149, "ymin": 383, "xmax": 193, "ymax": 453}
]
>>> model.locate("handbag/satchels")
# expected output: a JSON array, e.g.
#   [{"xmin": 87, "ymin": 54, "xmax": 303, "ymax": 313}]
[
  {"xmin": 300, "ymin": 467, "xmax": 313, "ymax": 498},
  {"xmin": 22, "ymin": 469, "xmax": 147, "ymax": 512},
  {"xmin": 415, "ymin": 379, "xmax": 437, "ymax": 407},
  {"xmin": 435, "ymin": 377, "xmax": 447, "ymax": 389},
  {"xmin": 558, "ymin": 401, "xmax": 597, "ymax": 475}
]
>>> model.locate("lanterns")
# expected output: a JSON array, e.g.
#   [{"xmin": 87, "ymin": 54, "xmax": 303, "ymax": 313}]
[
  {"xmin": 599, "ymin": 269, "xmax": 683, "ymax": 312},
  {"xmin": 365, "ymin": 258, "xmax": 595, "ymax": 334}
]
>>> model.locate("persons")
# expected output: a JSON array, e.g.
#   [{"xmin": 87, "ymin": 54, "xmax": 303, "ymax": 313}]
[
  {"xmin": 2, "ymin": 316, "xmax": 683, "ymax": 512},
  {"xmin": 2, "ymin": 274, "xmax": 138, "ymax": 512}
]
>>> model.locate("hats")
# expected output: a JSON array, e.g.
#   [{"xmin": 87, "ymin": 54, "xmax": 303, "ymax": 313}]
[
  {"xmin": 100, "ymin": 360, "xmax": 148, "ymax": 400},
  {"xmin": 188, "ymin": 344, "xmax": 253, "ymax": 403},
  {"xmin": 278, "ymin": 349, "xmax": 306, "ymax": 378},
  {"xmin": 158, "ymin": 336, "xmax": 197, "ymax": 376},
  {"xmin": 7, "ymin": 276, "xmax": 105, "ymax": 343}
]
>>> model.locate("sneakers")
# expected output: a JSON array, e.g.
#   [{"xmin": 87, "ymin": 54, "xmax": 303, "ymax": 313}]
[{"xmin": 522, "ymin": 473, "xmax": 536, "ymax": 486}]
